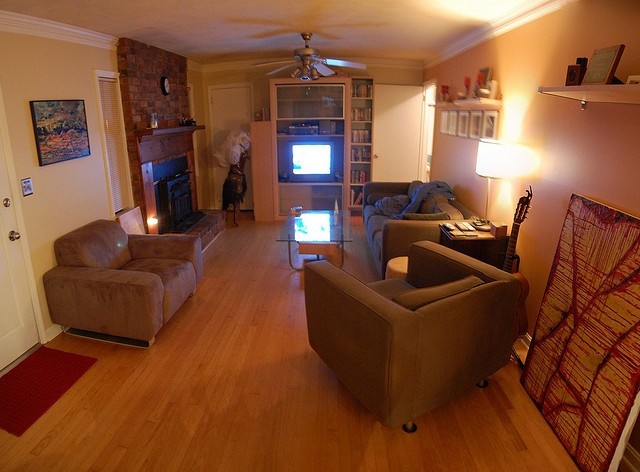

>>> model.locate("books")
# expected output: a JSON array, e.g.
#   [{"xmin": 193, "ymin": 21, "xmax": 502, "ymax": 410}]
[
  {"xmin": 351, "ymin": 188, "xmax": 366, "ymax": 208},
  {"xmin": 351, "ymin": 99, "xmax": 375, "ymax": 122},
  {"xmin": 349, "ymin": 122, "xmax": 374, "ymax": 145},
  {"xmin": 348, "ymin": 79, "xmax": 375, "ymax": 98},
  {"xmin": 349, "ymin": 162, "xmax": 370, "ymax": 183},
  {"xmin": 348, "ymin": 144, "xmax": 371, "ymax": 164}
]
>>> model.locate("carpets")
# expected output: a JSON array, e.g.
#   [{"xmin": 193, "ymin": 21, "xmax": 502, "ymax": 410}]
[{"xmin": 0, "ymin": 345, "xmax": 98, "ymax": 436}]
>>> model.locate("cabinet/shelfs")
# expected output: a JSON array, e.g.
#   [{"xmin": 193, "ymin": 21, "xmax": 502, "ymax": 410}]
[
  {"xmin": 435, "ymin": 98, "xmax": 499, "ymax": 110},
  {"xmin": 134, "ymin": 125, "xmax": 206, "ymax": 143},
  {"xmin": 350, "ymin": 76, "xmax": 376, "ymax": 212},
  {"xmin": 272, "ymin": 79, "xmax": 350, "ymax": 223},
  {"xmin": 538, "ymin": 83, "xmax": 640, "ymax": 110},
  {"xmin": 251, "ymin": 121, "xmax": 274, "ymax": 222}
]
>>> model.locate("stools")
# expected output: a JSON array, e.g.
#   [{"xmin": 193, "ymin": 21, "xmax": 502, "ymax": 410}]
[{"xmin": 384, "ymin": 256, "xmax": 409, "ymax": 282}]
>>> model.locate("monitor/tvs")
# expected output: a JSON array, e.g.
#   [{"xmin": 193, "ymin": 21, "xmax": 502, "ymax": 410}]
[
  {"xmin": 294, "ymin": 213, "xmax": 330, "ymax": 242},
  {"xmin": 292, "ymin": 144, "xmax": 331, "ymax": 174}
]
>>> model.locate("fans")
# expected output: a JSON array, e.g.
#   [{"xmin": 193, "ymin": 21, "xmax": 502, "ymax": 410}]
[{"xmin": 249, "ymin": 48, "xmax": 368, "ymax": 81}]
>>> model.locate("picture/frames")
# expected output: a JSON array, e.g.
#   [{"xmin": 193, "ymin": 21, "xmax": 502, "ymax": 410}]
[
  {"xmin": 29, "ymin": 99, "xmax": 91, "ymax": 167},
  {"xmin": 439, "ymin": 111, "xmax": 448, "ymax": 134},
  {"xmin": 519, "ymin": 193, "xmax": 639, "ymax": 472},
  {"xmin": 580, "ymin": 44, "xmax": 626, "ymax": 85},
  {"xmin": 468, "ymin": 109, "xmax": 483, "ymax": 139},
  {"xmin": 448, "ymin": 111, "xmax": 457, "ymax": 136},
  {"xmin": 480, "ymin": 110, "xmax": 499, "ymax": 143},
  {"xmin": 21, "ymin": 177, "xmax": 34, "ymax": 197},
  {"xmin": 456, "ymin": 109, "xmax": 469, "ymax": 138}
]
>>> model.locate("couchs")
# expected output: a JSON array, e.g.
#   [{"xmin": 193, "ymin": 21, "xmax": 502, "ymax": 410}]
[
  {"xmin": 44, "ymin": 219, "xmax": 203, "ymax": 350},
  {"xmin": 304, "ymin": 227, "xmax": 521, "ymax": 433},
  {"xmin": 362, "ymin": 180, "xmax": 479, "ymax": 280}
]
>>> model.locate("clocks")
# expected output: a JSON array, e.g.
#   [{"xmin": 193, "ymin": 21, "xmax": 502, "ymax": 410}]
[{"xmin": 160, "ymin": 76, "xmax": 171, "ymax": 95}]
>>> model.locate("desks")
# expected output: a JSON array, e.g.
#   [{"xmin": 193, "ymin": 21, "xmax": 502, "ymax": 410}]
[{"xmin": 441, "ymin": 221, "xmax": 508, "ymax": 271}]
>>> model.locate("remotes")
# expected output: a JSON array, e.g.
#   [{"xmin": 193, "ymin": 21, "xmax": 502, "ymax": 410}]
[
  {"xmin": 443, "ymin": 222, "xmax": 454, "ymax": 232},
  {"xmin": 463, "ymin": 221, "xmax": 476, "ymax": 232},
  {"xmin": 456, "ymin": 222, "xmax": 467, "ymax": 231}
]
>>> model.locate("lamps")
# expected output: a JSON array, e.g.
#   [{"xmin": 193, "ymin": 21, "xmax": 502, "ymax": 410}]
[
  {"xmin": 475, "ymin": 139, "xmax": 523, "ymax": 230},
  {"xmin": 290, "ymin": 67, "xmax": 300, "ymax": 79},
  {"xmin": 300, "ymin": 68, "xmax": 311, "ymax": 82},
  {"xmin": 310, "ymin": 69, "xmax": 320, "ymax": 80}
]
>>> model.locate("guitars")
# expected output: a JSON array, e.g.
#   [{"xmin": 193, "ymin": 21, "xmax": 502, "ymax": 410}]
[{"xmin": 503, "ymin": 185, "xmax": 530, "ymax": 346}]
